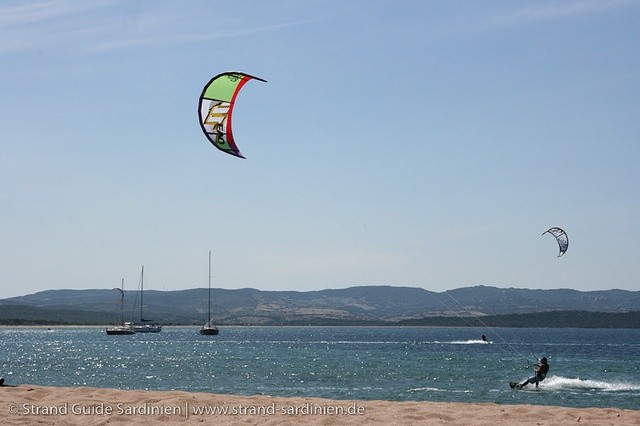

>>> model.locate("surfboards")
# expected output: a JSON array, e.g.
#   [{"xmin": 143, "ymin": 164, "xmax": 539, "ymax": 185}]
[{"xmin": 510, "ymin": 382, "xmax": 521, "ymax": 390}]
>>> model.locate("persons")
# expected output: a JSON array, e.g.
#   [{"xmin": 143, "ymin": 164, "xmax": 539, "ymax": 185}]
[
  {"xmin": 516, "ymin": 356, "xmax": 550, "ymax": 389},
  {"xmin": 481, "ymin": 336, "xmax": 488, "ymax": 341}
]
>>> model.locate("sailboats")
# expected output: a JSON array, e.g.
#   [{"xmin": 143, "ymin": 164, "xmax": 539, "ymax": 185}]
[
  {"xmin": 106, "ymin": 278, "xmax": 135, "ymax": 335},
  {"xmin": 199, "ymin": 250, "xmax": 219, "ymax": 335},
  {"xmin": 135, "ymin": 265, "xmax": 162, "ymax": 331}
]
